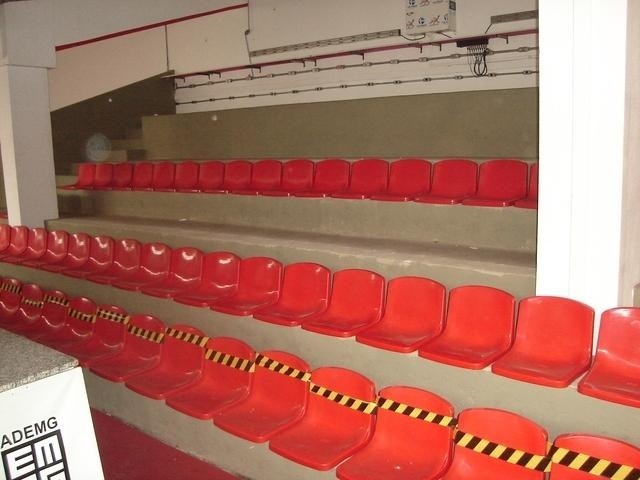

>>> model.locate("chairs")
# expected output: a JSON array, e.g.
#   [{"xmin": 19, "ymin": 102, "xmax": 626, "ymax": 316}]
[
  {"xmin": 549, "ymin": 433, "xmax": 638, "ymax": 479},
  {"xmin": 252, "ymin": 261, "xmax": 331, "ymax": 327},
  {"xmin": 491, "ymin": 295, "xmax": 593, "ymax": 389},
  {"xmin": 214, "ymin": 349, "xmax": 310, "ymax": 445},
  {"xmin": 418, "ymin": 284, "xmax": 514, "ymax": 369},
  {"xmin": 56, "ymin": 160, "xmax": 539, "ymax": 212},
  {"xmin": 355, "ymin": 276, "xmax": 445, "ymax": 353},
  {"xmin": 335, "ymin": 386, "xmax": 453, "ymax": 478},
  {"xmin": 1, "ymin": 276, "xmax": 164, "ymax": 383},
  {"xmin": 302, "ymin": 268, "xmax": 385, "ymax": 337},
  {"xmin": 126, "ymin": 325, "xmax": 206, "ymax": 402},
  {"xmin": 166, "ymin": 337, "xmax": 254, "ymax": 420},
  {"xmin": 1, "ymin": 222, "xmax": 170, "ymax": 291},
  {"xmin": 140, "ymin": 246, "xmax": 203, "ymax": 299},
  {"xmin": 441, "ymin": 409, "xmax": 549, "ymax": 479},
  {"xmin": 575, "ymin": 308, "xmax": 640, "ymax": 408},
  {"xmin": 175, "ymin": 252, "xmax": 242, "ymax": 308},
  {"xmin": 208, "ymin": 256, "xmax": 283, "ymax": 317},
  {"xmin": 267, "ymin": 366, "xmax": 377, "ymax": 471}
]
